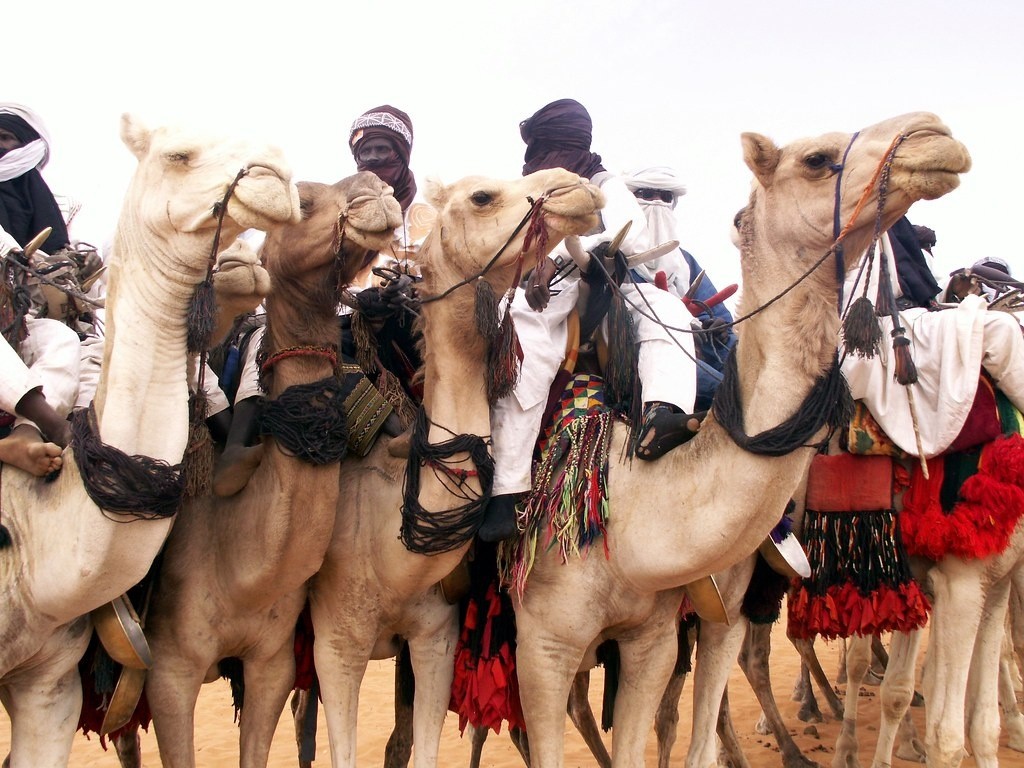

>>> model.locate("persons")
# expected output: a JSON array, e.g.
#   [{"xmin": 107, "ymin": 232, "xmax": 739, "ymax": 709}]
[
  {"xmin": 0, "ymin": 105, "xmax": 288, "ymax": 510},
  {"xmin": 731, "ymin": 203, "xmax": 988, "ymax": 351},
  {"xmin": 618, "ymin": 161, "xmax": 738, "ymax": 407},
  {"xmin": 474, "ymin": 100, "xmax": 714, "ymax": 543},
  {"xmin": 317, "ymin": 104, "xmax": 444, "ymax": 460}
]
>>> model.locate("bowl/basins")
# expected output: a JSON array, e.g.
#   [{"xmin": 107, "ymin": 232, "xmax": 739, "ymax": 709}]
[
  {"xmin": 760, "ymin": 530, "xmax": 812, "ymax": 579},
  {"xmin": 682, "ymin": 574, "xmax": 730, "ymax": 628},
  {"xmin": 93, "ymin": 594, "xmax": 152, "ymax": 672},
  {"xmin": 440, "ymin": 559, "xmax": 473, "ymax": 607},
  {"xmin": 99, "ymin": 658, "xmax": 145, "ymax": 736}
]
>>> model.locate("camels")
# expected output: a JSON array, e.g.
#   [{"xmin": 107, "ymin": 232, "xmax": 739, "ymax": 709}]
[{"xmin": 1, "ymin": 110, "xmax": 1024, "ymax": 768}]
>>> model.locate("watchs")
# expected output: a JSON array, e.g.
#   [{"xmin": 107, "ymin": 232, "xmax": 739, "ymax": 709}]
[{"xmin": 548, "ymin": 252, "xmax": 565, "ymax": 270}]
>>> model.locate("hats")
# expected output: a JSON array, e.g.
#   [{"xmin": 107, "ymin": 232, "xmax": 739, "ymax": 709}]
[
  {"xmin": 349, "ymin": 104, "xmax": 414, "ymax": 167},
  {"xmin": 623, "ymin": 162, "xmax": 685, "ymax": 209},
  {"xmin": 0, "ymin": 102, "xmax": 50, "ymax": 171},
  {"xmin": 973, "ymin": 256, "xmax": 1011, "ymax": 276},
  {"xmin": 519, "ymin": 98, "xmax": 592, "ymax": 151}
]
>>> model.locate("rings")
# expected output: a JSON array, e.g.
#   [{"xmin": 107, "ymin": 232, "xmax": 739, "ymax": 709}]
[
  {"xmin": 402, "ymin": 292, "xmax": 408, "ymax": 298},
  {"xmin": 533, "ymin": 284, "xmax": 540, "ymax": 288}
]
